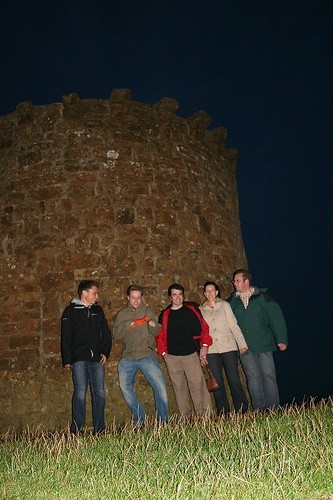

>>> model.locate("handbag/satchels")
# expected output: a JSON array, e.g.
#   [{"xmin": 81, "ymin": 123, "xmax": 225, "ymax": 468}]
[{"xmin": 202, "ymin": 365, "xmax": 219, "ymax": 392}]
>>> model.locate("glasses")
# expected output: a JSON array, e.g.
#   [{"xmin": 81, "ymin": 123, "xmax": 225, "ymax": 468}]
[{"xmin": 231, "ymin": 278, "xmax": 248, "ymax": 283}]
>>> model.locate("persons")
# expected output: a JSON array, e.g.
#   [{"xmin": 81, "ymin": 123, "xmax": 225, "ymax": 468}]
[
  {"xmin": 114, "ymin": 284, "xmax": 170, "ymax": 434},
  {"xmin": 60, "ymin": 279, "xmax": 113, "ymax": 436},
  {"xmin": 196, "ymin": 281, "xmax": 250, "ymax": 419},
  {"xmin": 153, "ymin": 283, "xmax": 213, "ymax": 422},
  {"xmin": 224, "ymin": 268, "xmax": 288, "ymax": 414}
]
